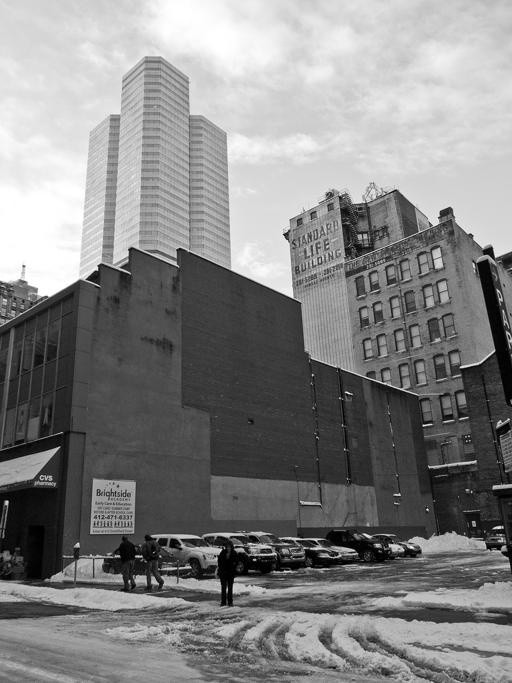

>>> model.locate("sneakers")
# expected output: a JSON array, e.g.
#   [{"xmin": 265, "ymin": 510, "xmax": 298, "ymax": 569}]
[
  {"xmin": 121, "ymin": 580, "xmax": 164, "ymax": 592},
  {"xmin": 220, "ymin": 600, "xmax": 234, "ymax": 607}
]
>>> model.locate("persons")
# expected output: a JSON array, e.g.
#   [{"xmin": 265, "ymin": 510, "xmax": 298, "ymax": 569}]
[
  {"xmin": 218, "ymin": 540, "xmax": 238, "ymax": 606},
  {"xmin": 111, "ymin": 534, "xmax": 164, "ymax": 591}
]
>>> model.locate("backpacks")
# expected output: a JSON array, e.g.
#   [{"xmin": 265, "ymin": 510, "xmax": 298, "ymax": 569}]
[{"xmin": 148, "ymin": 540, "xmax": 159, "ymax": 559}]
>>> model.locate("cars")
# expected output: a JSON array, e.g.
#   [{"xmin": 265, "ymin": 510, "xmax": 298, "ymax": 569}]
[
  {"xmin": 102, "ymin": 543, "xmax": 179, "ymax": 576},
  {"xmin": 279, "ymin": 529, "xmax": 423, "ymax": 575},
  {"xmin": 486, "ymin": 526, "xmax": 506, "ymax": 551}
]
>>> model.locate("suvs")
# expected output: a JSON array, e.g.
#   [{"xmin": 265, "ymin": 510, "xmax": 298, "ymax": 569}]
[
  {"xmin": 144, "ymin": 533, "xmax": 225, "ymax": 580},
  {"xmin": 201, "ymin": 531, "xmax": 278, "ymax": 576},
  {"xmin": 237, "ymin": 531, "xmax": 307, "ymax": 571}
]
